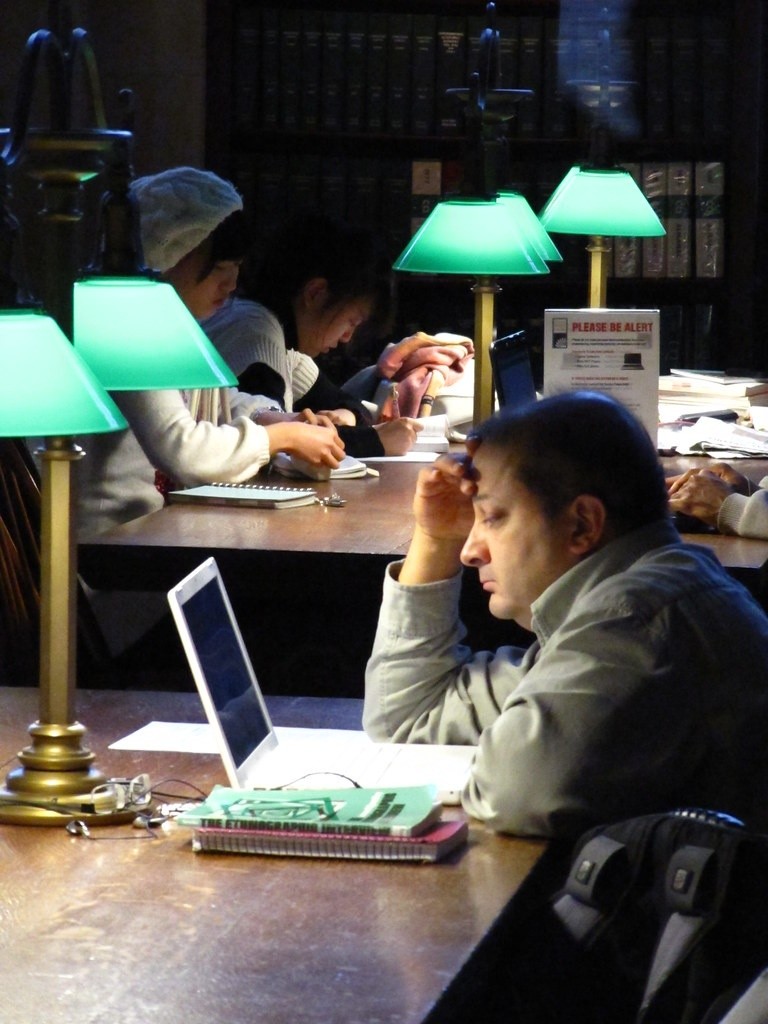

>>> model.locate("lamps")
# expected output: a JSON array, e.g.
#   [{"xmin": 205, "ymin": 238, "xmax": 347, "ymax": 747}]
[{"xmin": 0, "ymin": 165, "xmax": 670, "ymax": 825}]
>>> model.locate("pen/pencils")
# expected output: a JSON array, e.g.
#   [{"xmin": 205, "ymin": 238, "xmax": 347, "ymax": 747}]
[{"xmin": 392, "ymin": 387, "xmax": 400, "ymax": 421}]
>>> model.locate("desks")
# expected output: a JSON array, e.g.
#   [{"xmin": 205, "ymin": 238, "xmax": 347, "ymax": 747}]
[
  {"xmin": 79, "ymin": 418, "xmax": 768, "ymax": 585},
  {"xmin": 0, "ymin": 680, "xmax": 551, "ymax": 1024}
]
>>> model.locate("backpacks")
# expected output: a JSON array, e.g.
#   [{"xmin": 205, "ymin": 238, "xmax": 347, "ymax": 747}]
[{"xmin": 509, "ymin": 806, "xmax": 766, "ymax": 1024}]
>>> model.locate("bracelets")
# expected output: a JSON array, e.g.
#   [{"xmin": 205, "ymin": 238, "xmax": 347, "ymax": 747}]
[{"xmin": 270, "ymin": 406, "xmax": 283, "ymax": 411}]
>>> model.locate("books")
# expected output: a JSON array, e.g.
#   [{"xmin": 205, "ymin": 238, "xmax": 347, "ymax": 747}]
[
  {"xmin": 408, "ymin": 414, "xmax": 450, "ymax": 452},
  {"xmin": 168, "ymin": 482, "xmax": 317, "ymax": 509},
  {"xmin": 277, "ymin": 452, "xmax": 366, "ymax": 479},
  {"xmin": 208, "ymin": 0, "xmax": 727, "ymax": 370},
  {"xmin": 175, "ymin": 785, "xmax": 467, "ymax": 862},
  {"xmin": 659, "ymin": 369, "xmax": 767, "ymax": 418}
]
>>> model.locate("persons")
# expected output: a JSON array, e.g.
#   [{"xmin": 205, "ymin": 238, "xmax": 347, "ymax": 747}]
[
  {"xmin": 665, "ymin": 463, "xmax": 768, "ymax": 540},
  {"xmin": 362, "ymin": 391, "xmax": 768, "ymax": 835},
  {"xmin": 0, "ymin": 167, "xmax": 426, "ymax": 690}
]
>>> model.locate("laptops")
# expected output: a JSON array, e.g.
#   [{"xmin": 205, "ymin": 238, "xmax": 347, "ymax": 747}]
[
  {"xmin": 489, "ymin": 330, "xmax": 712, "ymax": 535},
  {"xmin": 167, "ymin": 556, "xmax": 479, "ymax": 804}
]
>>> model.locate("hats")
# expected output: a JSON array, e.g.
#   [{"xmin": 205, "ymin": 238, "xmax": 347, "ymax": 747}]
[{"xmin": 129, "ymin": 168, "xmax": 244, "ymax": 274}]
